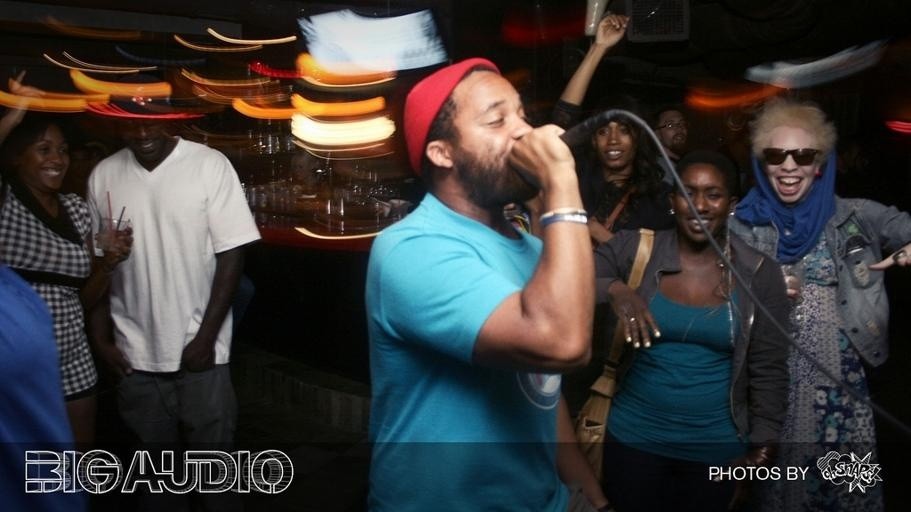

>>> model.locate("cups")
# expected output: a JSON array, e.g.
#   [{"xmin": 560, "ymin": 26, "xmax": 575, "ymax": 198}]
[
  {"xmin": 95, "ymin": 217, "xmax": 130, "ymax": 253},
  {"xmin": 238, "ymin": 112, "xmax": 397, "ymax": 231}
]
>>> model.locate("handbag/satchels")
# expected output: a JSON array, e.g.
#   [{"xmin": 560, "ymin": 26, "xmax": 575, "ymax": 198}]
[{"xmin": 563, "ymin": 370, "xmax": 617, "ymax": 512}]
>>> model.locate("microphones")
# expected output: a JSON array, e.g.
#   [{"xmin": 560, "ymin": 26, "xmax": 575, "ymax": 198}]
[{"xmin": 558, "ymin": 110, "xmax": 615, "ymax": 146}]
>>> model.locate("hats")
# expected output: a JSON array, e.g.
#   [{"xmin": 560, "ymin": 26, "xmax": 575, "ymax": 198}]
[{"xmin": 401, "ymin": 56, "xmax": 502, "ymax": 179}]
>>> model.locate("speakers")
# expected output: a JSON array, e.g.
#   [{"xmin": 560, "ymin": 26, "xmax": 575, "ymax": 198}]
[{"xmin": 625, "ymin": 0, "xmax": 690, "ymax": 42}]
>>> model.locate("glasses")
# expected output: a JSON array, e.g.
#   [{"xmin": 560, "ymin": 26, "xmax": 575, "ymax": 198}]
[
  {"xmin": 655, "ymin": 121, "xmax": 689, "ymax": 132},
  {"xmin": 758, "ymin": 144, "xmax": 825, "ymax": 168}
]
